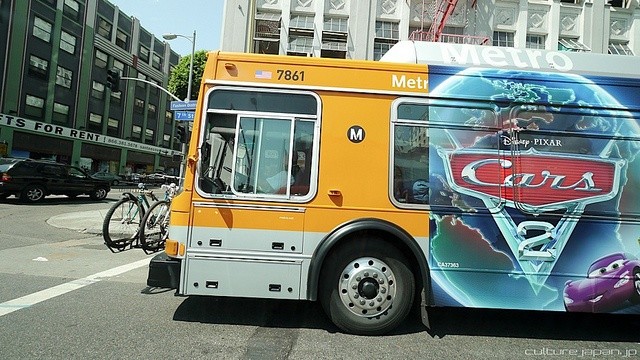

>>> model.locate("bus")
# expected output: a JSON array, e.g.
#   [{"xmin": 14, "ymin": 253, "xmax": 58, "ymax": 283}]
[{"xmin": 147, "ymin": 40, "xmax": 640, "ymax": 335}]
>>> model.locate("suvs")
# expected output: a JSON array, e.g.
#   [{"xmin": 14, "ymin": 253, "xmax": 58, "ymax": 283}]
[{"xmin": 0, "ymin": 156, "xmax": 111, "ymax": 203}]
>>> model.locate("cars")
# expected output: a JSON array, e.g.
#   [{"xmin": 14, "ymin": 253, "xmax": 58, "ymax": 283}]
[{"xmin": 76, "ymin": 172, "xmax": 121, "ymax": 185}]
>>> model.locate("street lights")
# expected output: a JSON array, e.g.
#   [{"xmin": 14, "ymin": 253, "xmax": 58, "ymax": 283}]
[{"xmin": 162, "ymin": 30, "xmax": 196, "ymax": 185}]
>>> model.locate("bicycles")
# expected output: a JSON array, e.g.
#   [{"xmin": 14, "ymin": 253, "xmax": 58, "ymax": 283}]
[
  {"xmin": 140, "ymin": 183, "xmax": 180, "ymax": 251},
  {"xmin": 103, "ymin": 183, "xmax": 170, "ymax": 248}
]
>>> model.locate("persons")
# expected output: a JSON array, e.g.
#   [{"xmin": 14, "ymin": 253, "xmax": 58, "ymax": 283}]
[
  {"xmin": 126, "ymin": 165, "xmax": 132, "ymax": 178},
  {"xmin": 414, "ymin": 179, "xmax": 429, "ymax": 202},
  {"xmin": 248, "ymin": 149, "xmax": 302, "ymax": 194}
]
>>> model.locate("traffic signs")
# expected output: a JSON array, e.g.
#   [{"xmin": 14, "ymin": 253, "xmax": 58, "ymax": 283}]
[
  {"xmin": 175, "ymin": 111, "xmax": 195, "ymax": 120},
  {"xmin": 170, "ymin": 100, "xmax": 197, "ymax": 110}
]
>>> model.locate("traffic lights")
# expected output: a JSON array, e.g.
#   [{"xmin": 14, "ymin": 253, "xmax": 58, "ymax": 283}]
[
  {"xmin": 177, "ymin": 125, "xmax": 187, "ymax": 142},
  {"xmin": 106, "ymin": 69, "xmax": 120, "ymax": 92}
]
